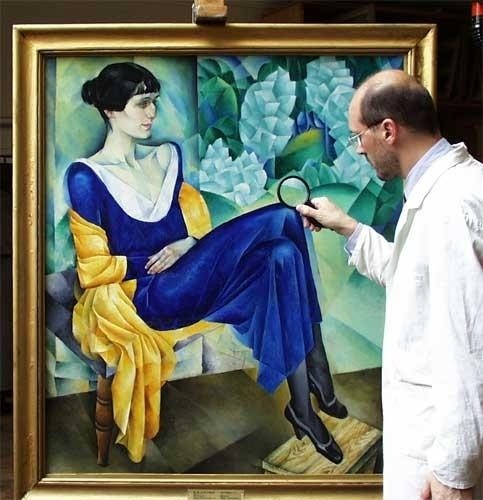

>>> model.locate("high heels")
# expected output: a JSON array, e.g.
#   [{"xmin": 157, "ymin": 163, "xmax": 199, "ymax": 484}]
[
  {"xmin": 283, "ymin": 398, "xmax": 345, "ymax": 466},
  {"xmin": 307, "ymin": 370, "xmax": 348, "ymax": 419}
]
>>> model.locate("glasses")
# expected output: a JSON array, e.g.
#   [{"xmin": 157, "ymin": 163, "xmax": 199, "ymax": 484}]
[{"xmin": 347, "ymin": 129, "xmax": 368, "ymax": 148}]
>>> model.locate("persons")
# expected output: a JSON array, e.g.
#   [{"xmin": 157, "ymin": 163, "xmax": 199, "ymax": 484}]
[
  {"xmin": 60, "ymin": 61, "xmax": 350, "ymax": 467},
  {"xmin": 296, "ymin": 66, "xmax": 483, "ymax": 500}
]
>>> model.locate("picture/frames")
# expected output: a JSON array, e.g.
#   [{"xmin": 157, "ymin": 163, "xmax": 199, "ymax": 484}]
[{"xmin": 14, "ymin": 25, "xmax": 447, "ymax": 500}]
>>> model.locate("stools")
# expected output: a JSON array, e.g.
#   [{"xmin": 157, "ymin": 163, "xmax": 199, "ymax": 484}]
[
  {"xmin": 261, "ymin": 407, "xmax": 382, "ymax": 474},
  {"xmin": 43, "ymin": 267, "xmax": 224, "ymax": 468}
]
